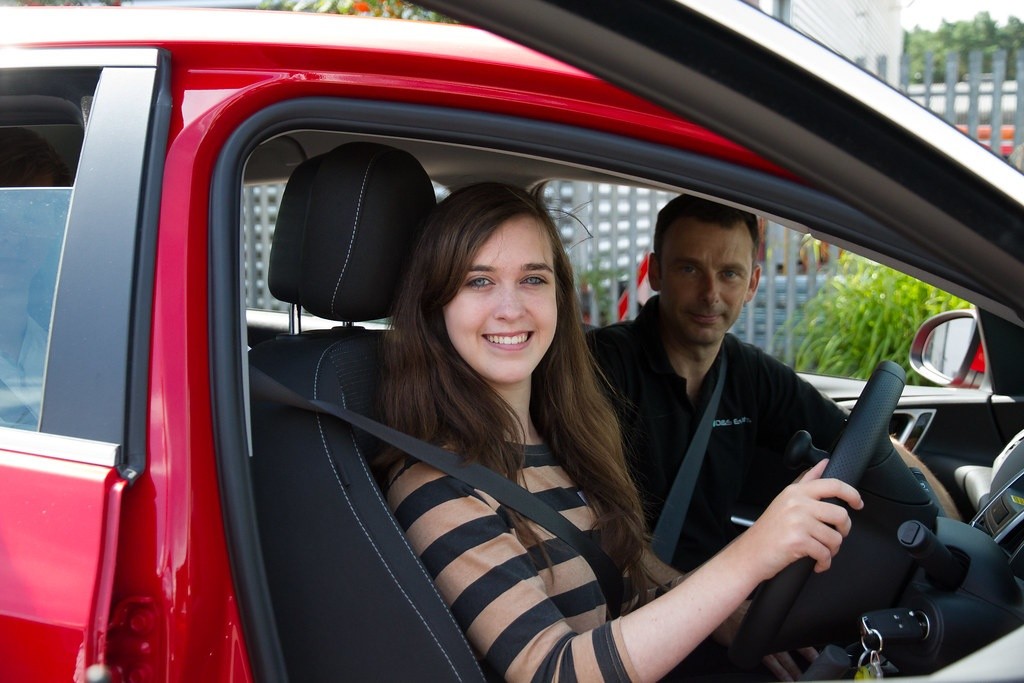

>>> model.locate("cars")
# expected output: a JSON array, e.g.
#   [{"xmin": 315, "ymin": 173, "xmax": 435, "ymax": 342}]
[{"xmin": 0, "ymin": 0, "xmax": 1024, "ymax": 683}]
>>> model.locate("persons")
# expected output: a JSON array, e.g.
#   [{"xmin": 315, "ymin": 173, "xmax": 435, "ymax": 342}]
[
  {"xmin": 0, "ymin": 126, "xmax": 71, "ymax": 432},
  {"xmin": 364, "ymin": 182, "xmax": 865, "ymax": 682},
  {"xmin": 585, "ymin": 194, "xmax": 961, "ymax": 682}
]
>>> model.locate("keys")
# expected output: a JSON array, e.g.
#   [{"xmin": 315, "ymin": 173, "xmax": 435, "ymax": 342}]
[{"xmin": 861, "ymin": 604, "xmax": 927, "ymax": 645}]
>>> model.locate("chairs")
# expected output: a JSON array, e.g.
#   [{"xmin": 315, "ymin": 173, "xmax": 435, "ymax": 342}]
[{"xmin": 248, "ymin": 141, "xmax": 497, "ymax": 683}]
